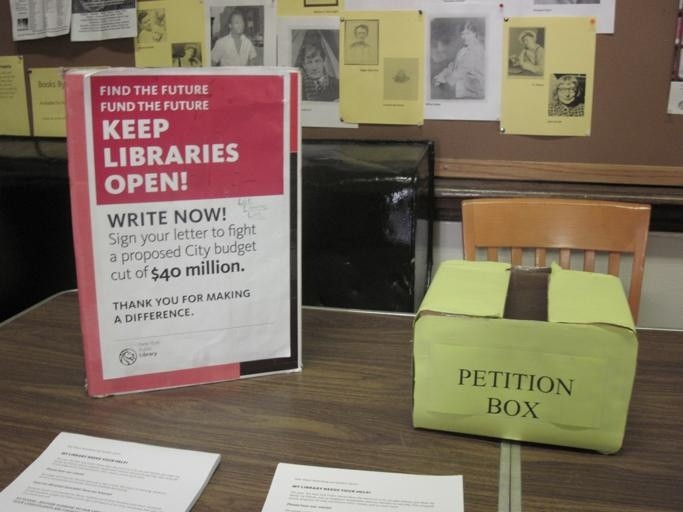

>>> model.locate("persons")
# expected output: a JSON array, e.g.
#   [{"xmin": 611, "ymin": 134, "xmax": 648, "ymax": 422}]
[
  {"xmin": 349, "ymin": 25, "xmax": 373, "ymax": 63},
  {"xmin": 549, "ymin": 74, "xmax": 583, "ymax": 117},
  {"xmin": 138, "ymin": 9, "xmax": 165, "ymax": 44},
  {"xmin": 519, "ymin": 29, "xmax": 545, "ymax": 76},
  {"xmin": 432, "ymin": 19, "xmax": 484, "ymax": 97},
  {"xmin": 170, "ymin": 44, "xmax": 201, "ymax": 66},
  {"xmin": 297, "ymin": 42, "xmax": 339, "ymax": 100},
  {"xmin": 211, "ymin": 12, "xmax": 257, "ymax": 65}
]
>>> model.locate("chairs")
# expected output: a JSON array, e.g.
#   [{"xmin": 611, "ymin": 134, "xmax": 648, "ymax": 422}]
[{"xmin": 460, "ymin": 199, "xmax": 651, "ymax": 330}]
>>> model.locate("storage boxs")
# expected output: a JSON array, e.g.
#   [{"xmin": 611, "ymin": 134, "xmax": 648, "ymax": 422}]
[{"xmin": 408, "ymin": 260, "xmax": 640, "ymax": 456}]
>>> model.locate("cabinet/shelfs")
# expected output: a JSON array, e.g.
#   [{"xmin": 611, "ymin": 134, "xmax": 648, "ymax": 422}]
[{"xmin": 0, "ymin": 131, "xmax": 436, "ymax": 315}]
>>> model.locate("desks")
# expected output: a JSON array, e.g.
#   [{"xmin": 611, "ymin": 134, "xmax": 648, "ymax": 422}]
[{"xmin": 0, "ymin": 290, "xmax": 683, "ymax": 511}]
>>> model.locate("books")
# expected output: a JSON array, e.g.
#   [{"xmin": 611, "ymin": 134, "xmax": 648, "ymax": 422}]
[{"xmin": 58, "ymin": 66, "xmax": 305, "ymax": 402}]
